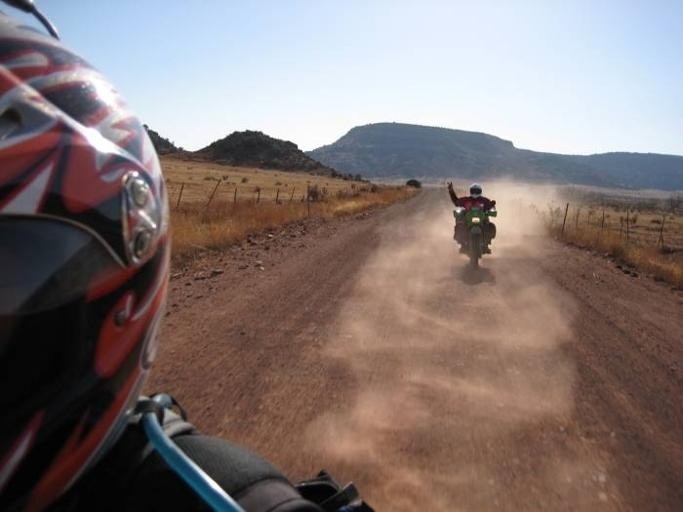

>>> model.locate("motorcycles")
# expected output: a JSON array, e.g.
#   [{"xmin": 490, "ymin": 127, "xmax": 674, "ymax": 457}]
[{"xmin": 454, "ymin": 201, "xmax": 499, "ymax": 267}]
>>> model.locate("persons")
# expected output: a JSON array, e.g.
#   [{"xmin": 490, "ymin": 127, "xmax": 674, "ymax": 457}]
[
  {"xmin": 446, "ymin": 179, "xmax": 497, "ymax": 255},
  {"xmin": 0, "ymin": 0, "xmax": 378, "ymax": 511}
]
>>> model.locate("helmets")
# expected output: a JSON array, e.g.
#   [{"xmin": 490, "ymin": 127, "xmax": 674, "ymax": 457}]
[
  {"xmin": 0, "ymin": 0, "xmax": 171, "ymax": 512},
  {"xmin": 470, "ymin": 183, "xmax": 482, "ymax": 199}
]
